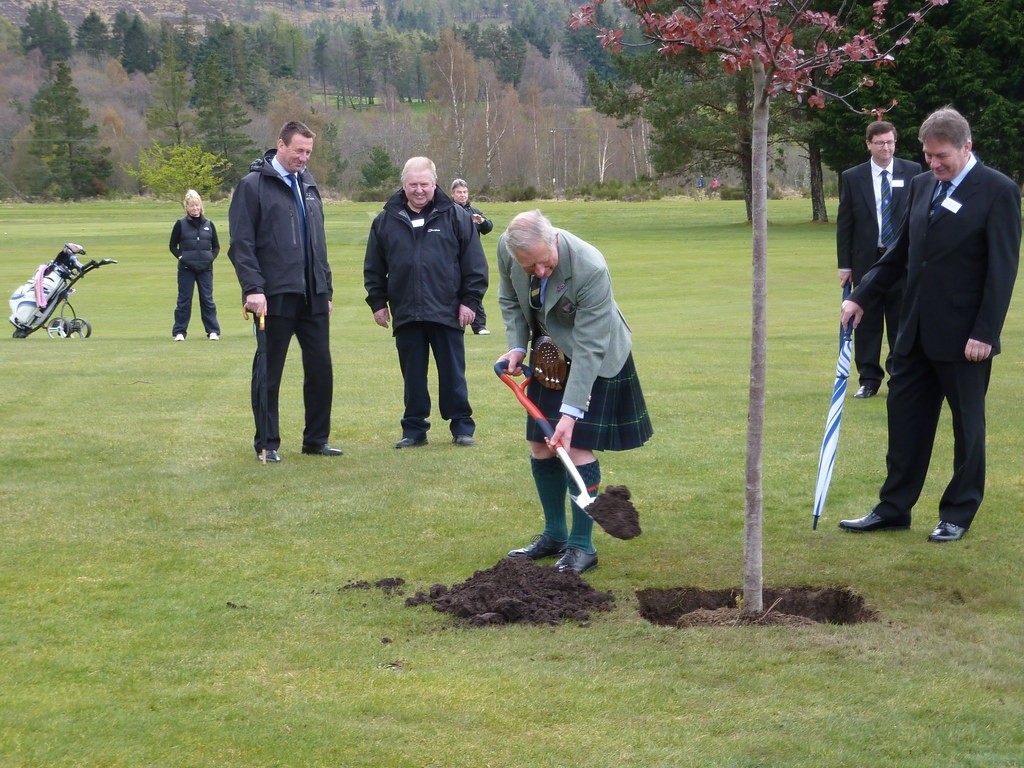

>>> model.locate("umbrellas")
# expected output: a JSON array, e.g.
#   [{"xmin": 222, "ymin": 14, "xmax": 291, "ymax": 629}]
[
  {"xmin": 839, "ymin": 280, "xmax": 852, "ymax": 351},
  {"xmin": 813, "ymin": 316, "xmax": 857, "ymax": 532}
]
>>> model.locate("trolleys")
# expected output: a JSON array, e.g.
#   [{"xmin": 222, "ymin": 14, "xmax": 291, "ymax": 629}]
[{"xmin": 8, "ymin": 242, "xmax": 118, "ymax": 338}]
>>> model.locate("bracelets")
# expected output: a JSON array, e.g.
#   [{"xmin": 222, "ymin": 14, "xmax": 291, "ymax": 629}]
[{"xmin": 562, "ymin": 413, "xmax": 578, "ymax": 420}]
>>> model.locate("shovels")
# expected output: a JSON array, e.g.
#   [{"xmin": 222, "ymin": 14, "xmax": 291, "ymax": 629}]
[{"xmin": 494, "ymin": 360, "xmax": 638, "ymax": 540}]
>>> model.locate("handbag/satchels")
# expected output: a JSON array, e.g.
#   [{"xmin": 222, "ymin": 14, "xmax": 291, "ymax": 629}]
[{"xmin": 531, "ymin": 335, "xmax": 568, "ymax": 390}]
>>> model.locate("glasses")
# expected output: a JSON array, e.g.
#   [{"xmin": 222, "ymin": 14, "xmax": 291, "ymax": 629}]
[{"xmin": 872, "ymin": 141, "xmax": 895, "ymax": 146}]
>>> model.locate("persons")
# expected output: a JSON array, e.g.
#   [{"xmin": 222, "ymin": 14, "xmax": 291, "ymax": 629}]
[
  {"xmin": 450, "ymin": 179, "xmax": 493, "ymax": 334},
  {"xmin": 497, "ymin": 209, "xmax": 654, "ymax": 575},
  {"xmin": 230, "ymin": 120, "xmax": 342, "ymax": 462},
  {"xmin": 169, "ymin": 189, "xmax": 221, "ymax": 340},
  {"xmin": 698, "ymin": 176, "xmax": 704, "ymax": 191},
  {"xmin": 837, "ymin": 121, "xmax": 922, "ymax": 398},
  {"xmin": 839, "ymin": 108, "xmax": 1023, "ymax": 543},
  {"xmin": 363, "ymin": 156, "xmax": 488, "ymax": 449},
  {"xmin": 712, "ymin": 178, "xmax": 720, "ymax": 196}
]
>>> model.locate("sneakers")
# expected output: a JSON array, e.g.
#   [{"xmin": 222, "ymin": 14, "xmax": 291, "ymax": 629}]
[
  {"xmin": 555, "ymin": 546, "xmax": 599, "ymax": 575},
  {"xmin": 508, "ymin": 534, "xmax": 568, "ymax": 560}
]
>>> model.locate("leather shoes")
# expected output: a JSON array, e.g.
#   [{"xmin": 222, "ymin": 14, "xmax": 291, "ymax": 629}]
[
  {"xmin": 840, "ymin": 510, "xmax": 910, "ymax": 533},
  {"xmin": 928, "ymin": 519, "xmax": 966, "ymax": 542},
  {"xmin": 257, "ymin": 449, "xmax": 280, "ymax": 463},
  {"xmin": 854, "ymin": 384, "xmax": 877, "ymax": 398},
  {"xmin": 302, "ymin": 445, "xmax": 344, "ymax": 456}
]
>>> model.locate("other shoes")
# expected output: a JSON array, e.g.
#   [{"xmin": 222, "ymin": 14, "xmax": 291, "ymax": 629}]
[
  {"xmin": 480, "ymin": 329, "xmax": 491, "ymax": 334},
  {"xmin": 210, "ymin": 332, "xmax": 219, "ymax": 340},
  {"xmin": 395, "ymin": 437, "xmax": 429, "ymax": 449},
  {"xmin": 453, "ymin": 435, "xmax": 474, "ymax": 445},
  {"xmin": 175, "ymin": 334, "xmax": 184, "ymax": 341}
]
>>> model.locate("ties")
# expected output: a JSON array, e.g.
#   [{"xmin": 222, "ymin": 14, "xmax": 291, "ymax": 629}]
[
  {"xmin": 531, "ymin": 275, "xmax": 543, "ymax": 309},
  {"xmin": 880, "ymin": 170, "xmax": 896, "ymax": 250},
  {"xmin": 928, "ymin": 181, "xmax": 953, "ymax": 221},
  {"xmin": 287, "ymin": 174, "xmax": 307, "ymax": 239}
]
